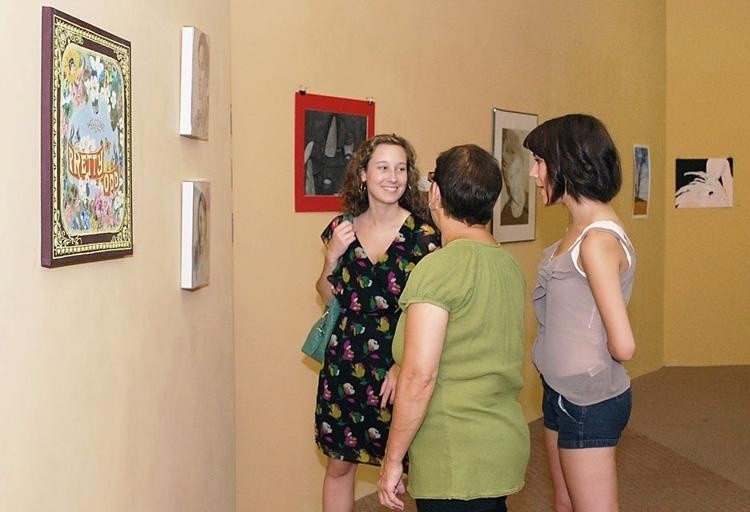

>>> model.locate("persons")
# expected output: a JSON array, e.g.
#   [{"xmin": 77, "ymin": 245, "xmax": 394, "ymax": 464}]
[
  {"xmin": 375, "ymin": 143, "xmax": 532, "ymax": 510},
  {"xmin": 520, "ymin": 112, "xmax": 636, "ymax": 510},
  {"xmin": 314, "ymin": 133, "xmax": 444, "ymax": 512},
  {"xmin": 500, "ymin": 128, "xmax": 530, "ymax": 226}
]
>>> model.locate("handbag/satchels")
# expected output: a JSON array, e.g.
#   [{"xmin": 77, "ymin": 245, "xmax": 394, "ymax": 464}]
[{"xmin": 301, "ymin": 300, "xmax": 341, "ymax": 362}]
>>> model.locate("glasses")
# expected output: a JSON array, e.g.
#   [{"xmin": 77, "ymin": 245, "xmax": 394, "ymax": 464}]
[{"xmin": 426, "ymin": 168, "xmax": 438, "ymax": 188}]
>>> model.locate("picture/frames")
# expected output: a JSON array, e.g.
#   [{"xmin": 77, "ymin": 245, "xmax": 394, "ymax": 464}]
[
  {"xmin": 492, "ymin": 107, "xmax": 539, "ymax": 245},
  {"xmin": 40, "ymin": 5, "xmax": 134, "ymax": 270},
  {"xmin": 295, "ymin": 93, "xmax": 375, "ymax": 214}
]
